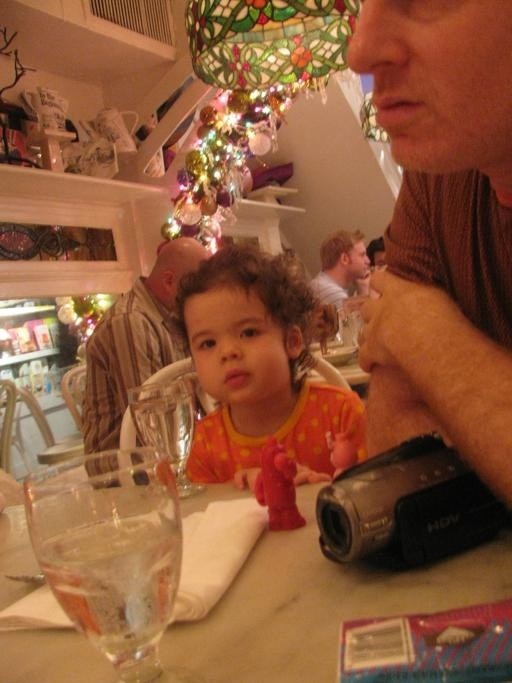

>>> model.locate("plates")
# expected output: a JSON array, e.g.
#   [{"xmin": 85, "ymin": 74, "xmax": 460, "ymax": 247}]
[{"xmin": 312, "ymin": 346, "xmax": 359, "ymax": 364}]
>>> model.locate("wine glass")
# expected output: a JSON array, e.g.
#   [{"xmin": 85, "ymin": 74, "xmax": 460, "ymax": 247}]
[{"xmin": 127, "ymin": 379, "xmax": 208, "ymax": 499}]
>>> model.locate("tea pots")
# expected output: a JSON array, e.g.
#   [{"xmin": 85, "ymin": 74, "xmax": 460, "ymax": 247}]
[
  {"xmin": 20, "ymin": 85, "xmax": 69, "ymax": 130},
  {"xmin": 77, "ymin": 105, "xmax": 139, "ymax": 152}
]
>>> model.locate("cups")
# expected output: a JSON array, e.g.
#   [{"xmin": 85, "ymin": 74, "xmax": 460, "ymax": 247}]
[
  {"xmin": 23, "ymin": 443, "xmax": 184, "ymax": 682},
  {"xmin": 335, "ymin": 310, "xmax": 359, "ymax": 344}
]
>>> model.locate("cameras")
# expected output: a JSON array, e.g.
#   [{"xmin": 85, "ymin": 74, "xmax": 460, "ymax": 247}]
[{"xmin": 315, "ymin": 433, "xmax": 512, "ymax": 571}]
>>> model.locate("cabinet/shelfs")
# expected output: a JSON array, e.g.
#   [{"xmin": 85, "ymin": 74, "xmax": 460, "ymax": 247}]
[{"xmin": 0, "ymin": 295, "xmax": 118, "ymax": 412}]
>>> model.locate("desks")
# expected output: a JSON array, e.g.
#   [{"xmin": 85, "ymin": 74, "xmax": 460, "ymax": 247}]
[{"xmin": 1, "ymin": 477, "xmax": 510, "ymax": 682}]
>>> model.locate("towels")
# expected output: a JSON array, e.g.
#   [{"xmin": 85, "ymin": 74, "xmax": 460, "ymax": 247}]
[{"xmin": 2, "ymin": 495, "xmax": 272, "ymax": 631}]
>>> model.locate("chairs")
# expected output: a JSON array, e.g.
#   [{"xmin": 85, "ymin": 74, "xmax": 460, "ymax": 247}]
[
  {"xmin": 112, "ymin": 345, "xmax": 358, "ymax": 483},
  {"xmin": 0, "ymin": 383, "xmax": 54, "ymax": 477},
  {"xmin": 61, "ymin": 364, "xmax": 87, "ymax": 433},
  {"xmin": 0, "ymin": 379, "xmax": 19, "ymax": 475}
]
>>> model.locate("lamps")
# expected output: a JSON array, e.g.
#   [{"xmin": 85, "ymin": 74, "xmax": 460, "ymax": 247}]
[{"xmin": 183, "ymin": 0, "xmax": 361, "ymax": 89}]
